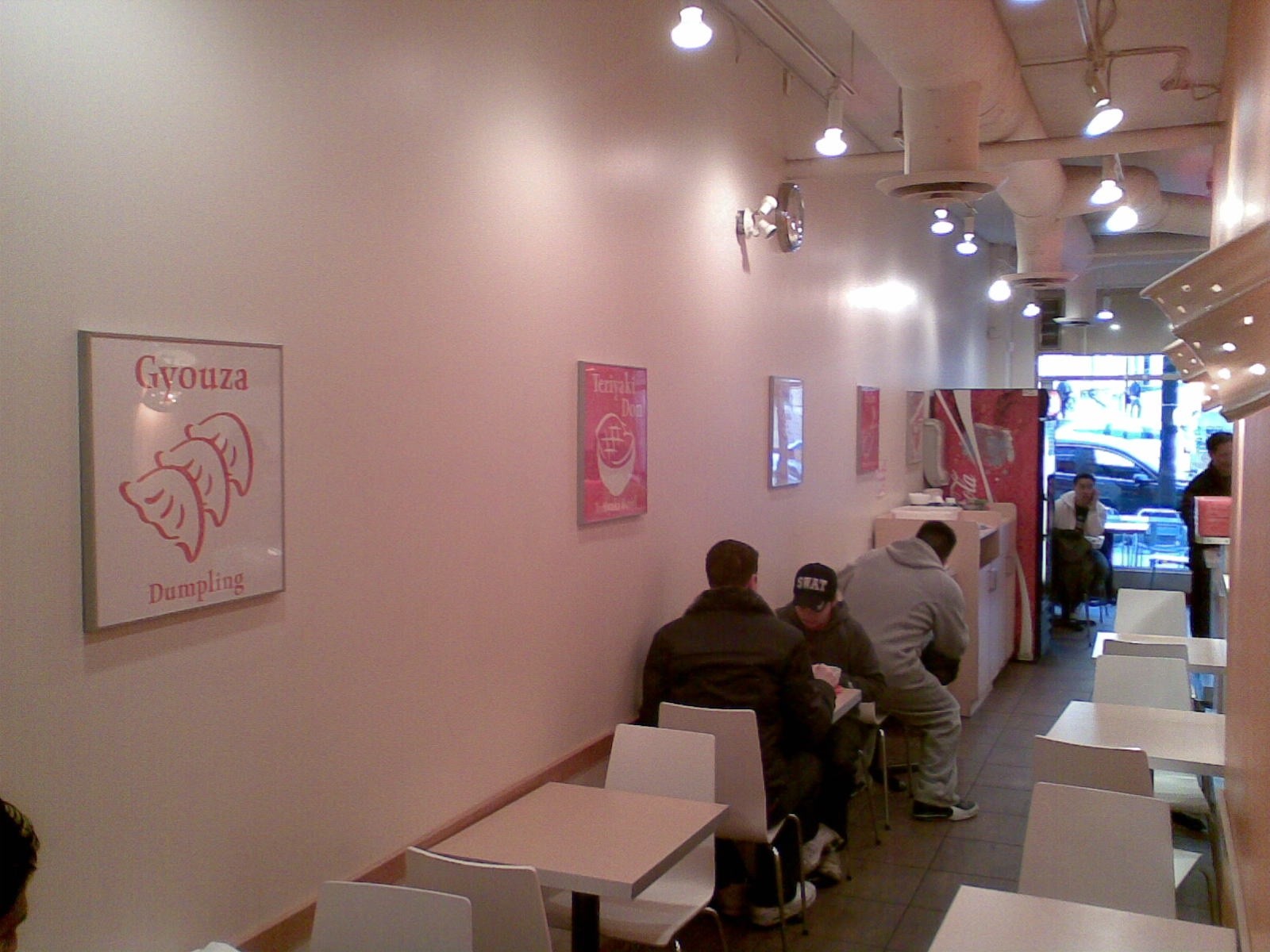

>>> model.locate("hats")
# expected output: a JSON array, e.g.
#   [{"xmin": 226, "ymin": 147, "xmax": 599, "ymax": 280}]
[{"xmin": 793, "ymin": 563, "xmax": 838, "ymax": 612}]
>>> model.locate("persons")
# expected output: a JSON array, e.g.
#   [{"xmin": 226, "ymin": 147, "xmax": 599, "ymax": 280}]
[
  {"xmin": 769, "ymin": 562, "xmax": 885, "ymax": 886},
  {"xmin": 638, "ymin": 539, "xmax": 817, "ymax": 926},
  {"xmin": 0, "ymin": 796, "xmax": 41, "ymax": 952},
  {"xmin": 1179, "ymin": 431, "xmax": 1235, "ymax": 639},
  {"xmin": 1048, "ymin": 473, "xmax": 1109, "ymax": 623},
  {"xmin": 832, "ymin": 519, "xmax": 981, "ymax": 820}
]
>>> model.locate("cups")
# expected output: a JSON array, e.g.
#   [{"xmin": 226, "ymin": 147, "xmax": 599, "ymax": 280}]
[{"xmin": 946, "ymin": 497, "xmax": 957, "ymax": 505}]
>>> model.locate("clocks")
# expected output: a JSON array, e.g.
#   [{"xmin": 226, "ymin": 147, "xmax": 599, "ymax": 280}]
[{"xmin": 774, "ymin": 182, "xmax": 805, "ymax": 254}]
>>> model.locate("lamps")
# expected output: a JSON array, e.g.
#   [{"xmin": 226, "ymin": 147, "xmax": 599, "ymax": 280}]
[
  {"xmin": 737, "ymin": 195, "xmax": 780, "ymax": 242},
  {"xmin": 956, "ymin": 216, "xmax": 979, "ymax": 254},
  {"xmin": 1084, "ymin": 69, "xmax": 1124, "ymax": 137},
  {"xmin": 1091, "ymin": 155, "xmax": 1123, "ymax": 204},
  {"xmin": 814, "ymin": 99, "xmax": 847, "ymax": 156},
  {"xmin": 670, "ymin": 0, "xmax": 713, "ymax": 50},
  {"xmin": 1096, "ymin": 296, "xmax": 1114, "ymax": 320}
]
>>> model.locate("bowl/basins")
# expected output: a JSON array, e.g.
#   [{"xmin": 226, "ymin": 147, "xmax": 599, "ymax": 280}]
[
  {"xmin": 964, "ymin": 504, "xmax": 986, "ymax": 511},
  {"xmin": 908, "ymin": 493, "xmax": 932, "ymax": 505},
  {"xmin": 922, "ymin": 488, "xmax": 945, "ymax": 503}
]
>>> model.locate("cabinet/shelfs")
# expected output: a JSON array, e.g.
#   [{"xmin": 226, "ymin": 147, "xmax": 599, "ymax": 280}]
[{"xmin": 876, "ymin": 502, "xmax": 1023, "ymax": 719}]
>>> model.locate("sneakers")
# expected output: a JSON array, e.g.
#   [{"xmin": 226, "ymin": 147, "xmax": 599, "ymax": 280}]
[
  {"xmin": 867, "ymin": 762, "xmax": 907, "ymax": 791},
  {"xmin": 910, "ymin": 798, "xmax": 980, "ymax": 821},
  {"xmin": 820, "ymin": 851, "xmax": 842, "ymax": 882},
  {"xmin": 753, "ymin": 882, "xmax": 817, "ymax": 926}
]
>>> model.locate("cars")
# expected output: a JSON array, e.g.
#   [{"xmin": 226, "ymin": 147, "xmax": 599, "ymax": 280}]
[{"xmin": 1055, "ymin": 438, "xmax": 1189, "ymax": 515}]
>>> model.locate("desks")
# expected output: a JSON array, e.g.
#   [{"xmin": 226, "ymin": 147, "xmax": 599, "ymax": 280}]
[
  {"xmin": 1104, "ymin": 521, "xmax": 1151, "ymax": 534},
  {"xmin": 427, "ymin": 782, "xmax": 733, "ymax": 952},
  {"xmin": 926, "ymin": 884, "xmax": 1242, "ymax": 952},
  {"xmin": 1092, "ymin": 632, "xmax": 1226, "ymax": 672},
  {"xmin": 1046, "ymin": 700, "xmax": 1227, "ymax": 794}
]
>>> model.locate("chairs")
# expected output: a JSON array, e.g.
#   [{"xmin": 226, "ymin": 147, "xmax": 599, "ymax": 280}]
[
  {"xmin": 312, "ymin": 700, "xmax": 896, "ymax": 952},
  {"xmin": 1017, "ymin": 588, "xmax": 1209, "ymax": 919}
]
